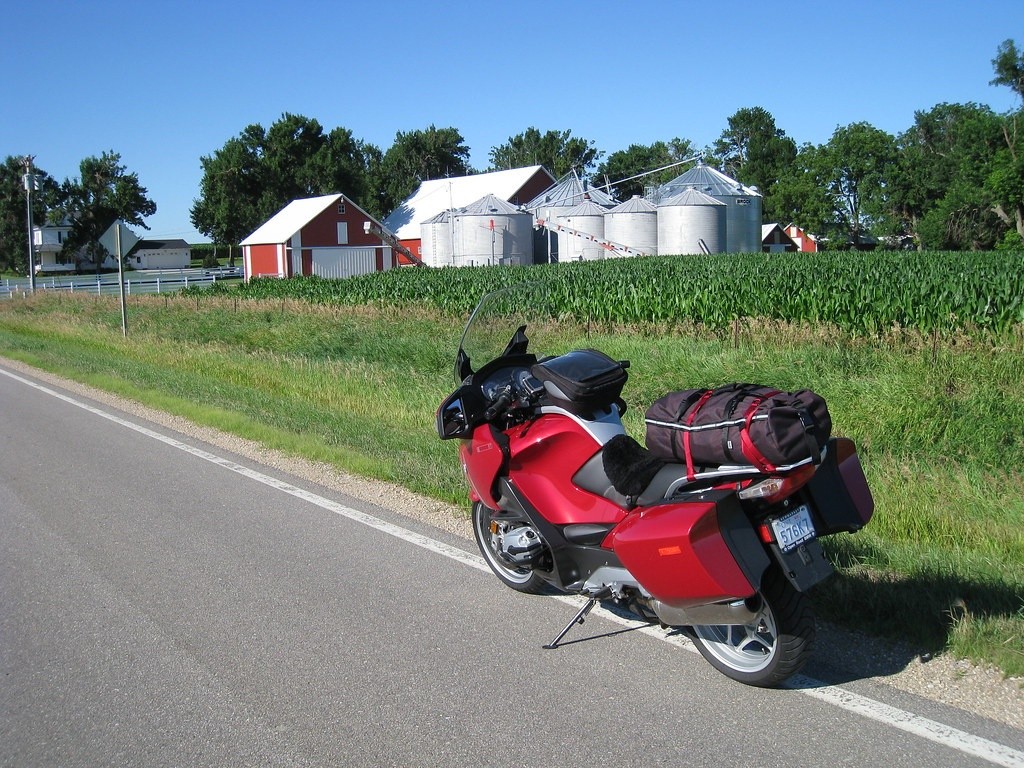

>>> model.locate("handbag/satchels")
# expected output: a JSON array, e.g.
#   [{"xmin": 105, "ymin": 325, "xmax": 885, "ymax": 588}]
[
  {"xmin": 644, "ymin": 383, "xmax": 832, "ymax": 466},
  {"xmin": 530, "ymin": 349, "xmax": 628, "ymax": 414}
]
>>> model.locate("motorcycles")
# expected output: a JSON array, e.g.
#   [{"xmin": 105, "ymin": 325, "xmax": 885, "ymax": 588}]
[{"xmin": 435, "ymin": 278, "xmax": 874, "ymax": 689}]
